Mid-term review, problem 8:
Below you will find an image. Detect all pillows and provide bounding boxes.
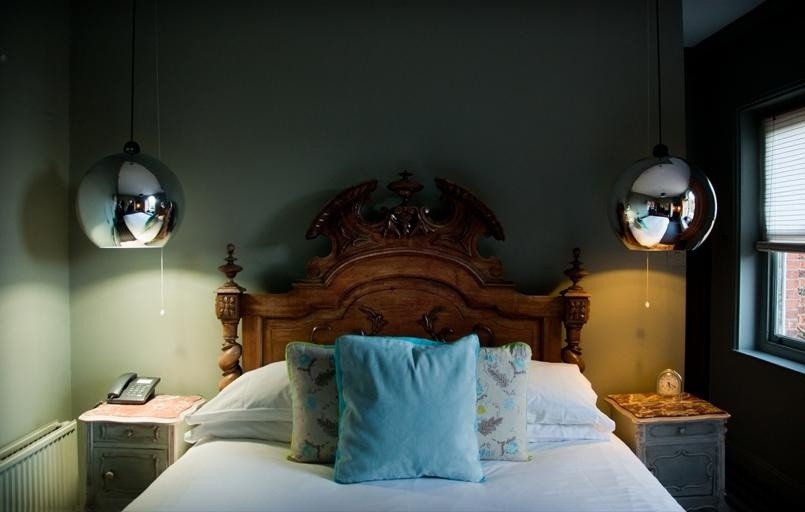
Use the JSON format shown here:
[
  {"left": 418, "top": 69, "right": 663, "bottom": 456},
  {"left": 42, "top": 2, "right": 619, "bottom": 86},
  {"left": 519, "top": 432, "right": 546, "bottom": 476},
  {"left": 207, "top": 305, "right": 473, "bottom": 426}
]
[
  {"left": 286, "top": 340, "right": 533, "bottom": 461},
  {"left": 184, "top": 423, "right": 291, "bottom": 444},
  {"left": 527, "top": 423, "right": 612, "bottom": 442},
  {"left": 183, "top": 360, "right": 294, "bottom": 425},
  {"left": 528, "top": 360, "right": 616, "bottom": 432},
  {"left": 331, "top": 333, "right": 486, "bottom": 484}
]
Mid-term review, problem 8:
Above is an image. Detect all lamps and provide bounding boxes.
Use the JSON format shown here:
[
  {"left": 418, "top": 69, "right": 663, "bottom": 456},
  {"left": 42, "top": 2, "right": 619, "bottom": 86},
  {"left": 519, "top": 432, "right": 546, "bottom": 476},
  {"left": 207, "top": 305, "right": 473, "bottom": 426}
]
[
  {"left": 607, "top": 1, "right": 717, "bottom": 252},
  {"left": 74, "top": 1, "right": 184, "bottom": 248}
]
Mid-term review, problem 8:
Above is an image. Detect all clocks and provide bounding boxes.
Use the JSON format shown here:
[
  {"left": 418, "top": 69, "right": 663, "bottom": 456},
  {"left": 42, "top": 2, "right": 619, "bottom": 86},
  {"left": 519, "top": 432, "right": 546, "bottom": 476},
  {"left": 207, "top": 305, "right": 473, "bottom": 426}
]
[{"left": 656, "top": 369, "right": 683, "bottom": 395}]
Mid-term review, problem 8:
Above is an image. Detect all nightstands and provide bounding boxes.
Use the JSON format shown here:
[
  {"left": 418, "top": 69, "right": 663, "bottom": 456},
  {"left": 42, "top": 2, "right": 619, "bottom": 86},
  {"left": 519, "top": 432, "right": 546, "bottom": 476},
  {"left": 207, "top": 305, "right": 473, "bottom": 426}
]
[
  {"left": 604, "top": 393, "right": 731, "bottom": 512},
  {"left": 78, "top": 395, "right": 206, "bottom": 512}
]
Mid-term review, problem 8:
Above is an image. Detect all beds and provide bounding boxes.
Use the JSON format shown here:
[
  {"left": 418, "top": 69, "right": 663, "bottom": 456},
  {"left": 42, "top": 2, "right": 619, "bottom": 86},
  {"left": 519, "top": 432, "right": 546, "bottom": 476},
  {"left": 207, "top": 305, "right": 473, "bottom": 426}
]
[{"left": 121, "top": 170, "right": 685, "bottom": 512}]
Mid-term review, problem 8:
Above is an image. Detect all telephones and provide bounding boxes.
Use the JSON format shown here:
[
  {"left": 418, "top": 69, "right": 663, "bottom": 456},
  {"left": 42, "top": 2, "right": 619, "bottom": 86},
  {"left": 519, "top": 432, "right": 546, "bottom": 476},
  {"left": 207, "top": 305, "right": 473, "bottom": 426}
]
[{"left": 105, "top": 372, "right": 160, "bottom": 404}]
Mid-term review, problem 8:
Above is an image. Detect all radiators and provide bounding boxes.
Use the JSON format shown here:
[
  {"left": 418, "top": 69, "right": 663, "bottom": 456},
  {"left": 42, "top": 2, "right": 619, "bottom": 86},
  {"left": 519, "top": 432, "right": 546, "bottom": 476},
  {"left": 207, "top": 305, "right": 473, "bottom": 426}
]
[{"left": 0, "top": 419, "right": 78, "bottom": 512}]
